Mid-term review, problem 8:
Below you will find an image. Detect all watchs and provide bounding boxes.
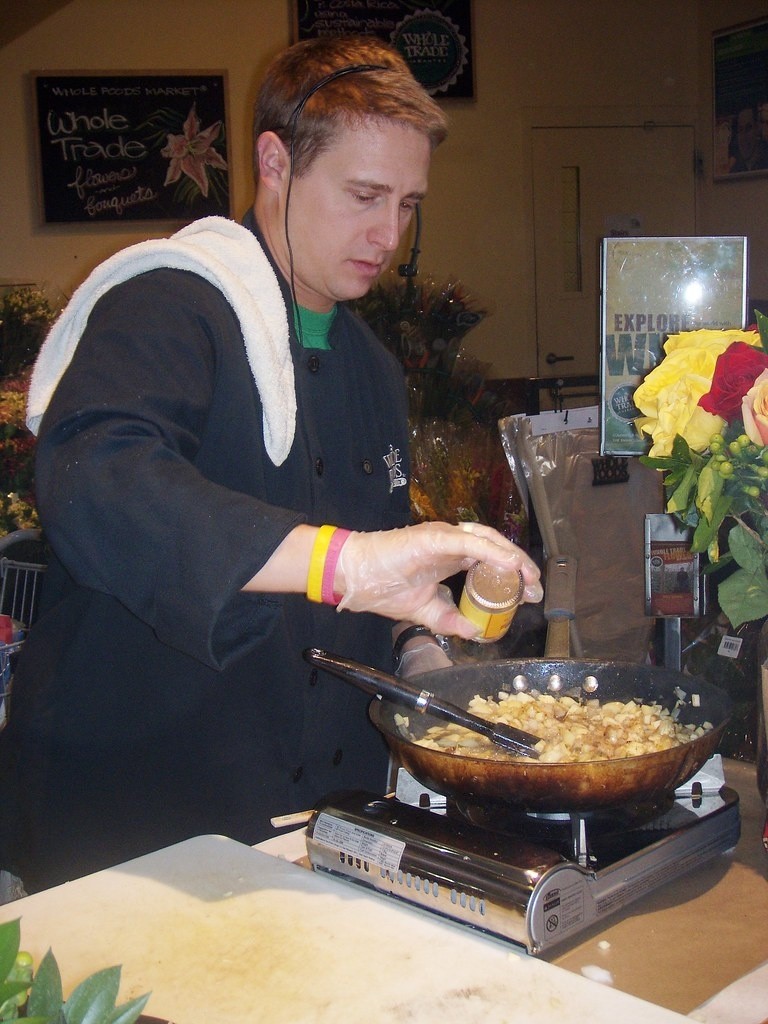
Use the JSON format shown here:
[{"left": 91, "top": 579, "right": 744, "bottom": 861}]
[{"left": 392, "top": 625, "right": 449, "bottom": 661}]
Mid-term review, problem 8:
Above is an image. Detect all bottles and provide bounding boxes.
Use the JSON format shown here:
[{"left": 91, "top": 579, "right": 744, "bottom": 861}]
[{"left": 458, "top": 559, "right": 524, "bottom": 645}]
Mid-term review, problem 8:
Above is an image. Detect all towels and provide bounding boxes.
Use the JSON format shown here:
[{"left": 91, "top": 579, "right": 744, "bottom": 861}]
[{"left": 26, "top": 214, "right": 297, "bottom": 467}]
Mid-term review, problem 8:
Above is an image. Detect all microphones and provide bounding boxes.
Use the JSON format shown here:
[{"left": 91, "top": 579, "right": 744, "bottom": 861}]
[{"left": 398, "top": 204, "right": 422, "bottom": 277}]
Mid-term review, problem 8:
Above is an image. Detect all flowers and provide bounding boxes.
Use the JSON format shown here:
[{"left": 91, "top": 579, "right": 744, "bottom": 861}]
[
  {"left": 0, "top": 278, "right": 77, "bottom": 537},
  {"left": 632, "top": 310, "right": 768, "bottom": 631},
  {"left": 348, "top": 273, "right": 528, "bottom": 550}
]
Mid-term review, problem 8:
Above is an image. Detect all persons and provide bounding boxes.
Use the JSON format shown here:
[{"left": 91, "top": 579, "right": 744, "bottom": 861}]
[{"left": 0, "top": 37, "right": 544, "bottom": 895}]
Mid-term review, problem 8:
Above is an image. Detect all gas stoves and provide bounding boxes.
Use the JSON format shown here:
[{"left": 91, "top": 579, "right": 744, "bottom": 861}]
[{"left": 305, "top": 753, "right": 742, "bottom": 956}]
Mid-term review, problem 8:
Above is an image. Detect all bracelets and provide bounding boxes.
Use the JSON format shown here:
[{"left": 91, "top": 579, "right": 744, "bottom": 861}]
[{"left": 307, "top": 525, "right": 352, "bottom": 605}]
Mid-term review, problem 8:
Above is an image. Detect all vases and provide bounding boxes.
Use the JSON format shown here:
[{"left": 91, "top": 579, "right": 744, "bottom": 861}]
[{"left": 756, "top": 620, "right": 768, "bottom": 803}]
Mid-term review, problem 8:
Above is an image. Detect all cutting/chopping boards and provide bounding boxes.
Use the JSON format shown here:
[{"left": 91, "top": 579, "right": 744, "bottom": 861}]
[{"left": 0, "top": 832, "right": 699, "bottom": 1024}]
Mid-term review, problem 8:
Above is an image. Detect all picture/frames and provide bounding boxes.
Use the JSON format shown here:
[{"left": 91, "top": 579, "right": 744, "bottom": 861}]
[{"left": 710, "top": 15, "right": 768, "bottom": 183}]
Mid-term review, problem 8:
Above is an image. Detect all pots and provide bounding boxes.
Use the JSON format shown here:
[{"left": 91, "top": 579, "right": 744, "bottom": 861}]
[{"left": 367, "top": 556, "right": 736, "bottom": 814}]
